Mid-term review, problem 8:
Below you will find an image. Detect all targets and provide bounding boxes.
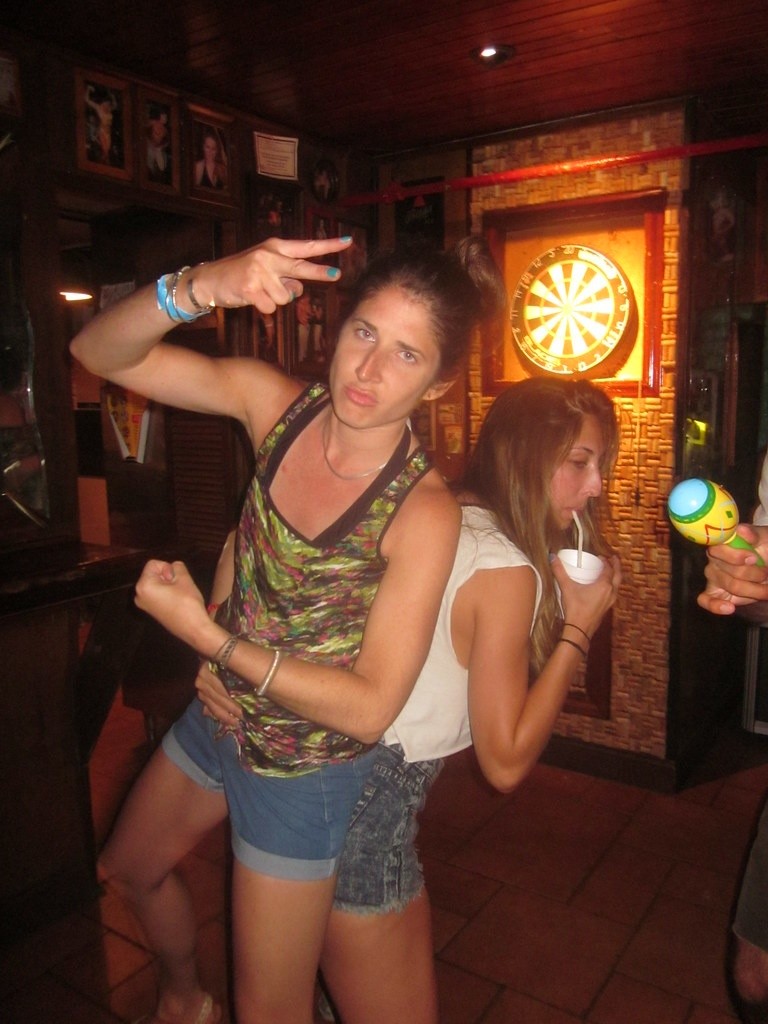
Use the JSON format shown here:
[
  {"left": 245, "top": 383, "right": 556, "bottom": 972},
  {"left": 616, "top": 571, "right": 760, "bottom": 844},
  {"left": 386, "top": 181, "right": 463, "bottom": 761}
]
[{"left": 509, "top": 241, "right": 633, "bottom": 376}]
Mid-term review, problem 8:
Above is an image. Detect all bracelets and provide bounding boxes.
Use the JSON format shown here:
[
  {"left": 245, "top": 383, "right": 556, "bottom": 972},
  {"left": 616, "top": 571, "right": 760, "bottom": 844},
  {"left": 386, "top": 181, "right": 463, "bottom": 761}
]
[
  {"left": 212, "top": 633, "right": 251, "bottom": 672},
  {"left": 252, "top": 648, "right": 284, "bottom": 698},
  {"left": 157, "top": 260, "right": 213, "bottom": 324},
  {"left": 560, "top": 622, "right": 593, "bottom": 646},
  {"left": 557, "top": 637, "right": 589, "bottom": 658}
]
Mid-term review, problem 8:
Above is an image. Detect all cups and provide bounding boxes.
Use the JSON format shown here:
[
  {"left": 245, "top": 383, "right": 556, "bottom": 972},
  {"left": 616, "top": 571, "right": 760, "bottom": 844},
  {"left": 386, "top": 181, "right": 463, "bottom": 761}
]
[{"left": 551, "top": 549, "right": 606, "bottom": 620}]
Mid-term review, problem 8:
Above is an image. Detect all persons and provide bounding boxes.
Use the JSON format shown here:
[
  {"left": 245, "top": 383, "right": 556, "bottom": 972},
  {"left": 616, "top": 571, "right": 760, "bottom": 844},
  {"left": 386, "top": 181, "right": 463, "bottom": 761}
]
[
  {"left": 84, "top": 81, "right": 126, "bottom": 168},
  {"left": 0, "top": 351, "right": 49, "bottom": 516},
  {"left": 695, "top": 440, "right": 768, "bottom": 1024},
  {"left": 252, "top": 155, "right": 421, "bottom": 374},
  {"left": 68, "top": 228, "right": 502, "bottom": 1023},
  {"left": 194, "top": 133, "right": 228, "bottom": 191},
  {"left": 145, "top": 110, "right": 174, "bottom": 178},
  {"left": 291, "top": 376, "right": 622, "bottom": 1024}
]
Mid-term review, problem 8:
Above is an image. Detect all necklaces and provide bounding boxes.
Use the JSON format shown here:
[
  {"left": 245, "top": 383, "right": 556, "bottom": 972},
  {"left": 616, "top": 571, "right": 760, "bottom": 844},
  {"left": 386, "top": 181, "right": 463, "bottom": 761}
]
[{"left": 322, "top": 401, "right": 408, "bottom": 479}]
